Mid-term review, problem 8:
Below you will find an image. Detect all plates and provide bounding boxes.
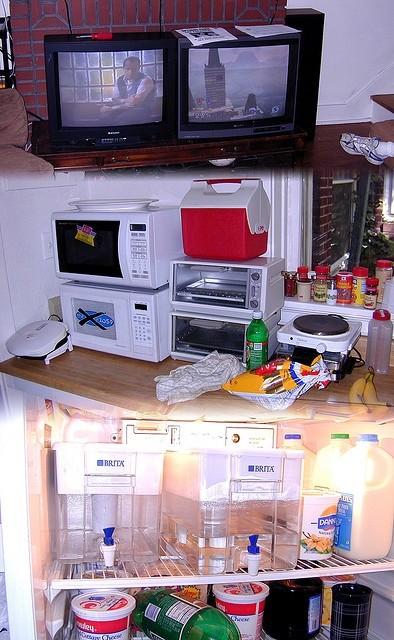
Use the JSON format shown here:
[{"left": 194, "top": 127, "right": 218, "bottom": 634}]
[{"left": 68, "top": 199, "right": 158, "bottom": 212}]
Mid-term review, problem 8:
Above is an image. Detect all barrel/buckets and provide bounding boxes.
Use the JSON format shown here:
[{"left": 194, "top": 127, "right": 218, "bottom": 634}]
[
  {"left": 331, "top": 433, "right": 394, "bottom": 561},
  {"left": 310, "top": 433, "right": 352, "bottom": 492},
  {"left": 279, "top": 435, "right": 315, "bottom": 488}
]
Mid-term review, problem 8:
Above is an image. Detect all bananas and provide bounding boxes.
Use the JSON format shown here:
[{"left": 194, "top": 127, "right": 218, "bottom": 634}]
[{"left": 349, "top": 366, "right": 390, "bottom": 415}]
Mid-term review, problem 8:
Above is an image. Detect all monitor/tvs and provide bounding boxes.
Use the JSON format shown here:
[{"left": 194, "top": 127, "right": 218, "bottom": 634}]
[
  {"left": 43, "top": 32, "right": 179, "bottom": 150},
  {"left": 171, "top": 25, "right": 301, "bottom": 141}
]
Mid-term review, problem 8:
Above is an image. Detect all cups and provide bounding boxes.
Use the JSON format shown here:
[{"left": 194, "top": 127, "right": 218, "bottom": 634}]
[{"left": 331, "top": 584, "right": 372, "bottom": 640}]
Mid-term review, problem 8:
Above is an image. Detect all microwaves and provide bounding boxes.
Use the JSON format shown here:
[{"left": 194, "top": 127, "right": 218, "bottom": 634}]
[
  {"left": 57, "top": 280, "right": 171, "bottom": 363},
  {"left": 51, "top": 206, "right": 184, "bottom": 290}
]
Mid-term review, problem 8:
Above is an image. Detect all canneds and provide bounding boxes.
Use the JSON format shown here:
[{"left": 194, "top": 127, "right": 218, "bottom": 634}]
[{"left": 313, "top": 275, "right": 326, "bottom": 302}]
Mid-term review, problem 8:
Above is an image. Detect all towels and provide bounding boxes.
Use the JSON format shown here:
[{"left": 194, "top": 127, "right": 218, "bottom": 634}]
[{"left": 155, "top": 349, "right": 243, "bottom": 406}]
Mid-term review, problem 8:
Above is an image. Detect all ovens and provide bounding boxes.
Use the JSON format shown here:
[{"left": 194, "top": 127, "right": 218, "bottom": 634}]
[
  {"left": 169, "top": 256, "right": 285, "bottom": 320},
  {"left": 167, "top": 310, "right": 281, "bottom": 367}
]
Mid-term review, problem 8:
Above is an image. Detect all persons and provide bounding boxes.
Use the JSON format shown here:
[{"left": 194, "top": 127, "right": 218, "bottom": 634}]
[{"left": 99, "top": 57, "right": 155, "bottom": 126}]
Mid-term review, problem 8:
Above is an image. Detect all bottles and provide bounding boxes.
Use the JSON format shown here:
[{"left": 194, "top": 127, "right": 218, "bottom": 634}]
[
  {"left": 314, "top": 275, "right": 327, "bottom": 302},
  {"left": 352, "top": 266, "right": 369, "bottom": 306},
  {"left": 246, "top": 311, "right": 269, "bottom": 370},
  {"left": 364, "top": 277, "right": 379, "bottom": 309},
  {"left": 365, "top": 309, "right": 393, "bottom": 374},
  {"left": 336, "top": 272, "right": 353, "bottom": 305},
  {"left": 375, "top": 260, "right": 393, "bottom": 309},
  {"left": 310, "top": 275, "right": 316, "bottom": 299},
  {"left": 284, "top": 272, "right": 296, "bottom": 296},
  {"left": 297, "top": 280, "right": 313, "bottom": 303},
  {"left": 298, "top": 266, "right": 309, "bottom": 279},
  {"left": 316, "top": 266, "right": 329, "bottom": 280},
  {"left": 327, "top": 277, "right": 338, "bottom": 304},
  {"left": 132, "top": 588, "right": 242, "bottom": 640}
]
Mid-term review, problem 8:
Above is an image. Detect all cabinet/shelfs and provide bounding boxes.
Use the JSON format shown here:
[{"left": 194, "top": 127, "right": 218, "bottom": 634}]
[
  {"left": 0, "top": 344, "right": 393, "bottom": 424},
  {"left": 372, "top": 95, "right": 393, "bottom": 179},
  {"left": 31, "top": 118, "right": 371, "bottom": 172}
]
